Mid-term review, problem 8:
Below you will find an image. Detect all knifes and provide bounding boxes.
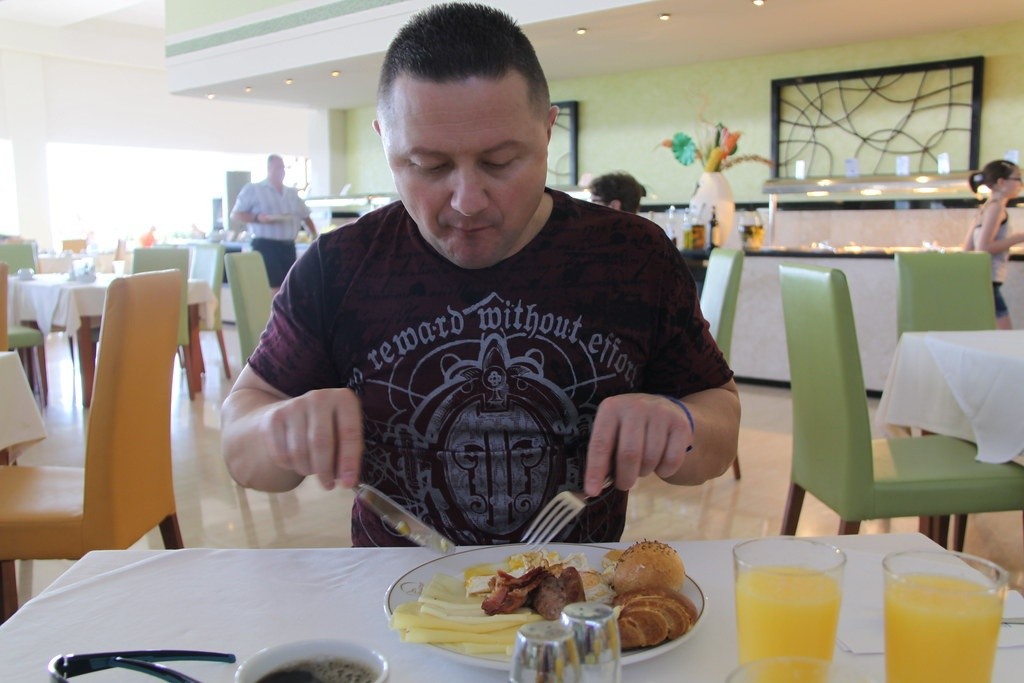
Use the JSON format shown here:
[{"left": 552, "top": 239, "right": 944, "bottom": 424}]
[{"left": 353, "top": 482, "right": 456, "bottom": 555}]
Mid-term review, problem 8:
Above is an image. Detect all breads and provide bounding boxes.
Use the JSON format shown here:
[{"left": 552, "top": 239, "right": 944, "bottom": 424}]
[{"left": 612, "top": 536, "right": 698, "bottom": 653}]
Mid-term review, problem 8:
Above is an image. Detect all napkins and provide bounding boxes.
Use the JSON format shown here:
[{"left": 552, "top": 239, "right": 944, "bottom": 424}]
[{"left": 828, "top": 586, "right": 1022, "bottom": 655}]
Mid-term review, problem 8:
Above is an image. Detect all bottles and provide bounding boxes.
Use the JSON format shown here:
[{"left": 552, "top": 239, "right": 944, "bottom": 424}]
[{"left": 666, "top": 204, "right": 720, "bottom": 250}]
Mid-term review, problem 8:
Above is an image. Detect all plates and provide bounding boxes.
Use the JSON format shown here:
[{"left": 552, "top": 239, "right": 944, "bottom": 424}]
[{"left": 384, "top": 542, "right": 705, "bottom": 671}]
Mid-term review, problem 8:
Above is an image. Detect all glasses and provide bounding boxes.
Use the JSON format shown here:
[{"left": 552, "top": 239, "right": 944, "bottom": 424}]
[
  {"left": 589, "top": 198, "right": 604, "bottom": 203},
  {"left": 1005, "top": 176, "right": 1023, "bottom": 182}
]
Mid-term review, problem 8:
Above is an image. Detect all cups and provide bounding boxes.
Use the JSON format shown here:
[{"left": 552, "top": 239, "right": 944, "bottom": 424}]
[
  {"left": 882, "top": 547, "right": 1007, "bottom": 682},
  {"left": 725, "top": 537, "right": 877, "bottom": 683},
  {"left": 738, "top": 209, "right": 765, "bottom": 249},
  {"left": 113, "top": 261, "right": 126, "bottom": 277}
]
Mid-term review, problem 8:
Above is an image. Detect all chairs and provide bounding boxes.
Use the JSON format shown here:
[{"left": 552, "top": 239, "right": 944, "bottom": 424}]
[
  {"left": 775, "top": 266, "right": 1024, "bottom": 551},
  {"left": 893, "top": 250, "right": 1001, "bottom": 344},
  {"left": 225, "top": 250, "right": 277, "bottom": 372},
  {"left": 0, "top": 265, "right": 186, "bottom": 623},
  {"left": 2, "top": 233, "right": 230, "bottom": 401},
  {"left": 700, "top": 243, "right": 744, "bottom": 481}
]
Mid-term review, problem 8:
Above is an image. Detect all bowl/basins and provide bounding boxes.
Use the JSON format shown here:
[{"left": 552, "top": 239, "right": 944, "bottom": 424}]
[{"left": 233, "top": 636, "right": 390, "bottom": 683}]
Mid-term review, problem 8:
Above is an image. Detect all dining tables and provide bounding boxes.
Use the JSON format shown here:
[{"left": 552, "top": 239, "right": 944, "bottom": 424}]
[
  {"left": 874, "top": 330, "right": 1023, "bottom": 556},
  {"left": 2, "top": 532, "right": 1022, "bottom": 683}
]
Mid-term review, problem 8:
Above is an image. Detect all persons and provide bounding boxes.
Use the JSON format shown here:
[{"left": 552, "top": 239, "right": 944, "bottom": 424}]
[
  {"left": 228, "top": 152, "right": 322, "bottom": 306},
  {"left": 584, "top": 171, "right": 648, "bottom": 218},
  {"left": 957, "top": 158, "right": 1023, "bottom": 331},
  {"left": 216, "top": 1, "right": 742, "bottom": 550}
]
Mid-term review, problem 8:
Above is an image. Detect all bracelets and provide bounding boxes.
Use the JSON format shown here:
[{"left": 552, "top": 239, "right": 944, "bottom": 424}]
[
  {"left": 658, "top": 395, "right": 696, "bottom": 435},
  {"left": 254, "top": 213, "right": 260, "bottom": 224}
]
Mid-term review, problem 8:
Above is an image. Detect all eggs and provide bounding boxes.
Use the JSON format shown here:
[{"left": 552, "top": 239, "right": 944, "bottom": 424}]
[{"left": 464, "top": 549, "right": 563, "bottom": 598}]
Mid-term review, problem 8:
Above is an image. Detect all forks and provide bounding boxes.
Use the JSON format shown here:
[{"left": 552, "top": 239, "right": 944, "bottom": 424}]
[{"left": 520, "top": 445, "right": 692, "bottom": 553}]
